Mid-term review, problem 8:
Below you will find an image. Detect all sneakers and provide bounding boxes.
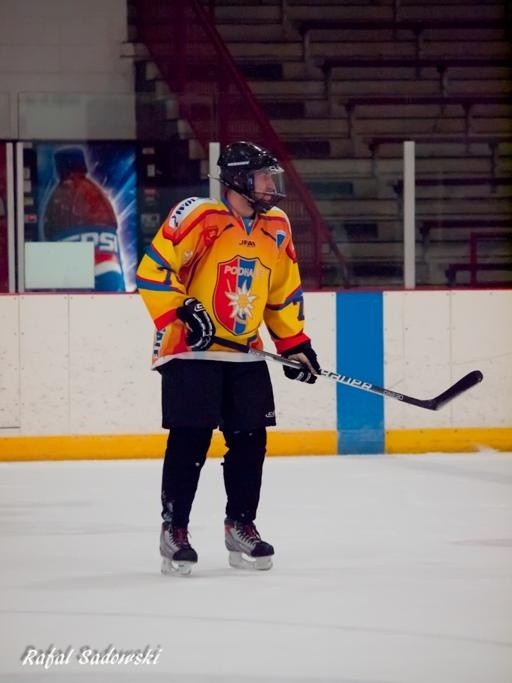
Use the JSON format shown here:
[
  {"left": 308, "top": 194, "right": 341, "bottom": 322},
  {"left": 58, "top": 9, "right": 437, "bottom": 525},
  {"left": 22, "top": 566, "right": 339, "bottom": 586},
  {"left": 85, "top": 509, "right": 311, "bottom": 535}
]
[
  {"left": 160, "top": 521, "right": 198, "bottom": 563},
  {"left": 224, "top": 518, "right": 274, "bottom": 557}
]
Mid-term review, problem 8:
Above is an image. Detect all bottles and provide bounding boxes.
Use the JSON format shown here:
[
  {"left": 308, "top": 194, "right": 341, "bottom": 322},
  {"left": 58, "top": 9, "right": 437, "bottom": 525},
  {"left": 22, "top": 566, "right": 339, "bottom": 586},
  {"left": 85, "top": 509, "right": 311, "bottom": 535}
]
[{"left": 39, "top": 145, "right": 129, "bottom": 291}]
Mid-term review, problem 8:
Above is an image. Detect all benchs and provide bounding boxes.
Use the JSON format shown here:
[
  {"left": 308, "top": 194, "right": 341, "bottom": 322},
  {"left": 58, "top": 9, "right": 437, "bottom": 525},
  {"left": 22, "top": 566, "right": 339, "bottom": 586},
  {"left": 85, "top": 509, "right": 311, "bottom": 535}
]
[{"left": 140, "top": 0, "right": 512, "bottom": 286}]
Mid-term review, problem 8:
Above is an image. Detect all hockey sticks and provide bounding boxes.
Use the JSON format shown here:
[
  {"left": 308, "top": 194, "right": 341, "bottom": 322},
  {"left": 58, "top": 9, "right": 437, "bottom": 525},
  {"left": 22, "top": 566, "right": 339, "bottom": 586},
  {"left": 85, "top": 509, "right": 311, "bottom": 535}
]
[{"left": 215, "top": 336, "right": 485, "bottom": 410}]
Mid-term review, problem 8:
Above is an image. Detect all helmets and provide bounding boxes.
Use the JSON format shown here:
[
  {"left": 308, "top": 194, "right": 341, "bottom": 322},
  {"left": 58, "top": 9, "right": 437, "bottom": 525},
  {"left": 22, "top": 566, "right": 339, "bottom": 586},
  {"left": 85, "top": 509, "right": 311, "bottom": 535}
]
[{"left": 216, "top": 141, "right": 289, "bottom": 214}]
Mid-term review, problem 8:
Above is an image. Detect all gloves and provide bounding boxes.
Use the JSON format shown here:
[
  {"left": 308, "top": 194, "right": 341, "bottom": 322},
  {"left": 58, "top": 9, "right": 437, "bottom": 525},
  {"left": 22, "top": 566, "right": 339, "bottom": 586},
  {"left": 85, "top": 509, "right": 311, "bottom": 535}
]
[
  {"left": 281, "top": 341, "right": 323, "bottom": 384},
  {"left": 175, "top": 297, "right": 215, "bottom": 352}
]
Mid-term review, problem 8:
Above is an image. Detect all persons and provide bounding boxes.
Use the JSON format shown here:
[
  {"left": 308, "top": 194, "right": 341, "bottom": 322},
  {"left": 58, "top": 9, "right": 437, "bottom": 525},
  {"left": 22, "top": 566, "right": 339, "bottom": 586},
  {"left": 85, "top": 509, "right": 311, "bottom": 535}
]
[{"left": 136, "top": 137, "right": 321, "bottom": 563}]
